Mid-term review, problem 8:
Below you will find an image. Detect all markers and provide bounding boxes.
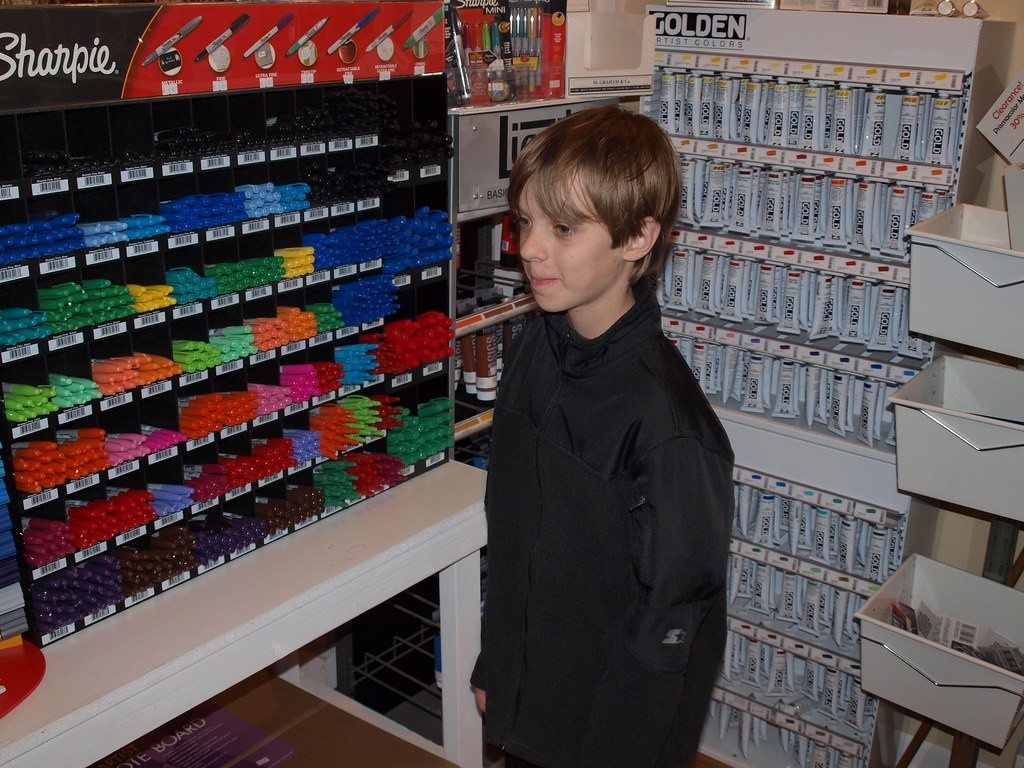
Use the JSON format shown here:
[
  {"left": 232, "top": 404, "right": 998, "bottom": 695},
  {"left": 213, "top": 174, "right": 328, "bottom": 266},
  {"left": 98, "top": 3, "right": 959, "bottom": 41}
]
[
  {"left": 1, "top": 82, "right": 462, "bottom": 646},
  {"left": 194, "top": 11, "right": 249, "bottom": 64},
  {"left": 142, "top": 14, "right": 203, "bottom": 69},
  {"left": 328, "top": 7, "right": 380, "bottom": 56},
  {"left": 284, "top": 16, "right": 330, "bottom": 57},
  {"left": 365, "top": 9, "right": 413, "bottom": 54},
  {"left": 244, "top": 12, "right": 294, "bottom": 59},
  {"left": 403, "top": 10, "right": 442, "bottom": 50}
]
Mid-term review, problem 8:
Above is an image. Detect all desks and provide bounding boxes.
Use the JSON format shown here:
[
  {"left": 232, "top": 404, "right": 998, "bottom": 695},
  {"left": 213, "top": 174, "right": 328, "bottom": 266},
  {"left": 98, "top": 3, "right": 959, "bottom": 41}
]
[{"left": 0, "top": 459, "right": 488, "bottom": 768}]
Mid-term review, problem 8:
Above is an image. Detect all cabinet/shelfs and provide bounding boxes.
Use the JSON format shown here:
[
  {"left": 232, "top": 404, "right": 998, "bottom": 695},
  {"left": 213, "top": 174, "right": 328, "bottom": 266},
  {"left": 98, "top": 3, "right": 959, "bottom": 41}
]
[
  {"left": 0, "top": 70, "right": 458, "bottom": 647},
  {"left": 646, "top": 5, "right": 1016, "bottom": 768},
  {"left": 450, "top": 93, "right": 619, "bottom": 442}
]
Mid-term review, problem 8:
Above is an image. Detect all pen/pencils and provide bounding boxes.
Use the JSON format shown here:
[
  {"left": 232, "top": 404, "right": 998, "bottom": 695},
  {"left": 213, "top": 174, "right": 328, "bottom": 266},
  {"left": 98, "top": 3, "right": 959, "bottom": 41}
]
[{"left": 449, "top": 7, "right": 549, "bottom": 102}]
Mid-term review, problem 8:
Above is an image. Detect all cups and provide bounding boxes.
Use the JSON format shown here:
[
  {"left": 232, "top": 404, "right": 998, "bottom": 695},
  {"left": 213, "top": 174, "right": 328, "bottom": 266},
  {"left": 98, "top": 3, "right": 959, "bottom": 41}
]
[{"left": 446, "top": 66, "right": 552, "bottom": 108}]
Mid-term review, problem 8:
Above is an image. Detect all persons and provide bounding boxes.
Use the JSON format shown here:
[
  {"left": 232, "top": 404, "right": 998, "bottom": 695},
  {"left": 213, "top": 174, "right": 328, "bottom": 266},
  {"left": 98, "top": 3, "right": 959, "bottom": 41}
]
[{"left": 471, "top": 106, "right": 735, "bottom": 768}]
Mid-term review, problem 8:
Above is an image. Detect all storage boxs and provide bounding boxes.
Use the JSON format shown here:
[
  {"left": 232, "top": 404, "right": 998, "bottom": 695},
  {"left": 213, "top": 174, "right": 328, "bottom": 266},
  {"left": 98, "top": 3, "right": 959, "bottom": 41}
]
[
  {"left": 855, "top": 202, "right": 1024, "bottom": 750},
  {"left": 444, "top": 0, "right": 568, "bottom": 110}
]
